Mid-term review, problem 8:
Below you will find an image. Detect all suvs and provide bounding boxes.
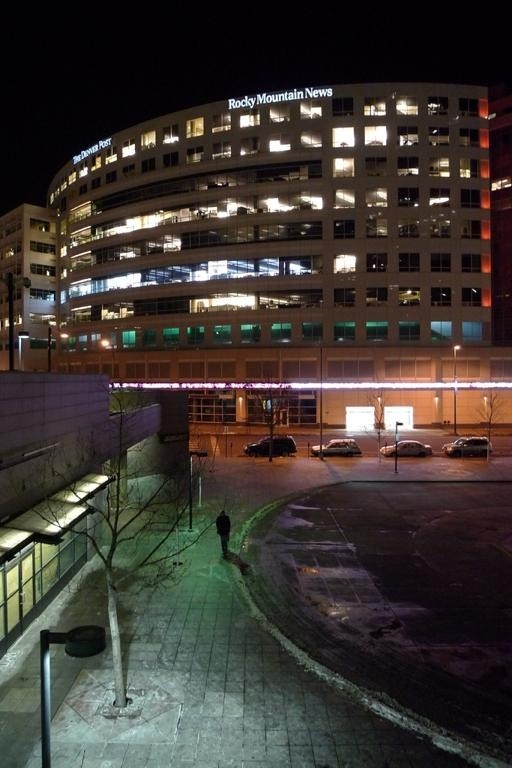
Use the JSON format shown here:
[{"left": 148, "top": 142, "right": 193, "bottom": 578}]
[
  {"left": 439, "top": 433, "right": 495, "bottom": 459},
  {"left": 242, "top": 433, "right": 298, "bottom": 458}
]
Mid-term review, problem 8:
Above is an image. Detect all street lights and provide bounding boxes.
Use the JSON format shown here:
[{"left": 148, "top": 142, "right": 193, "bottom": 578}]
[
  {"left": 37, "top": 620, "right": 112, "bottom": 765},
  {"left": 451, "top": 344, "right": 462, "bottom": 437},
  {"left": 315, "top": 335, "right": 345, "bottom": 460},
  {"left": 182, "top": 448, "right": 211, "bottom": 534},
  {"left": 393, "top": 420, "right": 403, "bottom": 473},
  {"left": 16, "top": 330, "right": 32, "bottom": 372}
]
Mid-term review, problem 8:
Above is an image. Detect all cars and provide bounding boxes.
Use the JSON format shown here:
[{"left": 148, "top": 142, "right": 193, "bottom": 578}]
[
  {"left": 380, "top": 436, "right": 433, "bottom": 459},
  {"left": 311, "top": 435, "right": 362, "bottom": 458}
]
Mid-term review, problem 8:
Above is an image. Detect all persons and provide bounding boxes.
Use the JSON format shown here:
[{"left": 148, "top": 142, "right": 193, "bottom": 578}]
[{"left": 216, "top": 511, "right": 231, "bottom": 557}]
